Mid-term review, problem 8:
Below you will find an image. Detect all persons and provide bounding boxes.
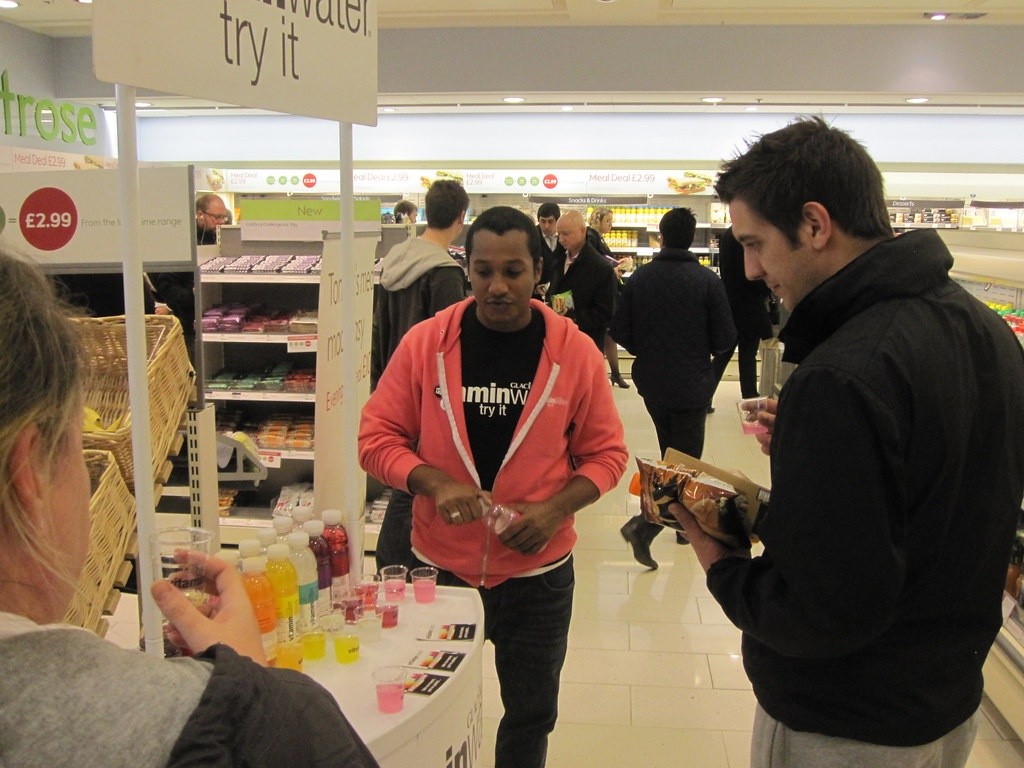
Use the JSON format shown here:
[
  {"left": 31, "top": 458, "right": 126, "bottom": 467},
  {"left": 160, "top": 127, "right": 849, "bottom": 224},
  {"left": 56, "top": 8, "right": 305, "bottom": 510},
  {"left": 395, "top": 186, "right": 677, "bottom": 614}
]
[
  {"left": 668, "top": 114, "right": 1024, "bottom": 768},
  {"left": 52, "top": 195, "right": 233, "bottom": 335},
  {"left": 719, "top": 225, "right": 780, "bottom": 399},
  {"left": 586, "top": 207, "right": 630, "bottom": 389},
  {"left": 358, "top": 206, "right": 629, "bottom": 768},
  {"left": 608, "top": 207, "right": 738, "bottom": 569},
  {"left": 0, "top": 250, "right": 382, "bottom": 768},
  {"left": 530, "top": 203, "right": 567, "bottom": 299},
  {"left": 370, "top": 180, "right": 470, "bottom": 582},
  {"left": 545, "top": 209, "right": 618, "bottom": 354},
  {"left": 393, "top": 199, "right": 417, "bottom": 224}
]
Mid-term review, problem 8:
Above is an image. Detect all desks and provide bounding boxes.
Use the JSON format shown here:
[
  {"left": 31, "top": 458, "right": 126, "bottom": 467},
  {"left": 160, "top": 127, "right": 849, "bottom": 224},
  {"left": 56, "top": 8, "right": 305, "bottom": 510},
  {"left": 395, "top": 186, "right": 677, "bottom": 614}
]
[{"left": 302, "top": 583, "right": 483, "bottom": 768}]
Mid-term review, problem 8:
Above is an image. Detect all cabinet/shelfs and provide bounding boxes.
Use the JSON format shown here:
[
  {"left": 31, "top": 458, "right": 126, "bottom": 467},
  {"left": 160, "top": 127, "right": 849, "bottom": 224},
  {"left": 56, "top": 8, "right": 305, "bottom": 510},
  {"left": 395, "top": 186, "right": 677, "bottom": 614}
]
[
  {"left": 581, "top": 200, "right": 763, "bottom": 360},
  {"left": 155, "top": 222, "right": 475, "bottom": 557}
]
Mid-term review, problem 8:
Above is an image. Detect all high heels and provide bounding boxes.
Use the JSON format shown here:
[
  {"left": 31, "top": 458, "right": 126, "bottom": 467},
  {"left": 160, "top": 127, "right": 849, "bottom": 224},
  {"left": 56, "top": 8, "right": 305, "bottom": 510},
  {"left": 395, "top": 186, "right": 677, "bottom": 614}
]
[{"left": 610, "top": 373, "right": 630, "bottom": 388}]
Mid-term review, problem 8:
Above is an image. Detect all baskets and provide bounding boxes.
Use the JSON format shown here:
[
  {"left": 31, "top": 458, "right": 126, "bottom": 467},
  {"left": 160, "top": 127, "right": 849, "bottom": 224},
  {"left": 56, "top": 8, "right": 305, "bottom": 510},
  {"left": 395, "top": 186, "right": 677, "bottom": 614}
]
[
  {"left": 60, "top": 449, "right": 137, "bottom": 632},
  {"left": 62, "top": 313, "right": 196, "bottom": 490}
]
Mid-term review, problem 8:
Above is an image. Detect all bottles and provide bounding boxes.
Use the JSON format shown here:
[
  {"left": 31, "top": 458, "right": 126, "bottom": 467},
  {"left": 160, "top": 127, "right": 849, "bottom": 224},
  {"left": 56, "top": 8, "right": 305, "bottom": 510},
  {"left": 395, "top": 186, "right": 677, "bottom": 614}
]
[
  {"left": 234, "top": 505, "right": 352, "bottom": 666},
  {"left": 477, "top": 498, "right": 547, "bottom": 555},
  {"left": 697, "top": 256, "right": 711, "bottom": 268},
  {"left": 612, "top": 254, "right": 653, "bottom": 272},
  {"left": 586, "top": 205, "right": 676, "bottom": 248},
  {"left": 980, "top": 299, "right": 1024, "bottom": 348}
]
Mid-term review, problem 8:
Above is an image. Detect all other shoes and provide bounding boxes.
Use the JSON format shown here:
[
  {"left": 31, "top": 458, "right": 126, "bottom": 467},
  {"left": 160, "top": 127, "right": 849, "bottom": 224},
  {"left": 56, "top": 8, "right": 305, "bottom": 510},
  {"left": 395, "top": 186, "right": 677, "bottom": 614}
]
[{"left": 706, "top": 406, "right": 716, "bottom": 413}]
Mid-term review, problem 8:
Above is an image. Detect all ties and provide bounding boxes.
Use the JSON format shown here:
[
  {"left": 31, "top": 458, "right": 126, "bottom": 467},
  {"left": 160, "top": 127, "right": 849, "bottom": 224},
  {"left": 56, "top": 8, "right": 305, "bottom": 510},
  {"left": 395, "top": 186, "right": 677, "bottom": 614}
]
[{"left": 547, "top": 235, "right": 556, "bottom": 252}]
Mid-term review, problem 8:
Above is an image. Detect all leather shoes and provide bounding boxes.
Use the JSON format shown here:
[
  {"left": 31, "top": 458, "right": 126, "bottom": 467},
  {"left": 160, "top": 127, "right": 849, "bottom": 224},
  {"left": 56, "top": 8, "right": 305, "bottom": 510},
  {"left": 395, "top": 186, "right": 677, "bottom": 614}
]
[
  {"left": 620, "top": 517, "right": 658, "bottom": 569},
  {"left": 677, "top": 530, "right": 689, "bottom": 545}
]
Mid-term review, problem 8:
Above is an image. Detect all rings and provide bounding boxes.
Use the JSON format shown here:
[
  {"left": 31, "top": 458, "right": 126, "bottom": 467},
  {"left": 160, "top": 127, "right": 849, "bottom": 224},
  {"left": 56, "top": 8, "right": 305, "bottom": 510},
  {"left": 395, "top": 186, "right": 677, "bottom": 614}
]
[{"left": 450, "top": 512, "right": 461, "bottom": 518}]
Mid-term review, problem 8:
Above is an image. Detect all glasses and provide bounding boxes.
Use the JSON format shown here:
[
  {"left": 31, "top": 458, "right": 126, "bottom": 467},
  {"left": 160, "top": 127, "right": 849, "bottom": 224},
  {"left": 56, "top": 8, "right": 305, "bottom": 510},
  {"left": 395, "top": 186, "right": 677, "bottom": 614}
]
[{"left": 202, "top": 210, "right": 229, "bottom": 222}]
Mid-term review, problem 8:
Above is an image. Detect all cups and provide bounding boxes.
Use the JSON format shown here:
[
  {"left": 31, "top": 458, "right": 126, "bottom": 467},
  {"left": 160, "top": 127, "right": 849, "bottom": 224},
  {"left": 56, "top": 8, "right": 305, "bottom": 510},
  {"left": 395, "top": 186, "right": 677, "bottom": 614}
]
[
  {"left": 379, "top": 565, "right": 409, "bottom": 602},
  {"left": 410, "top": 566, "right": 438, "bottom": 603},
  {"left": 372, "top": 666, "right": 408, "bottom": 714},
  {"left": 273, "top": 573, "right": 401, "bottom": 673},
  {"left": 734, "top": 395, "right": 769, "bottom": 436},
  {"left": 149, "top": 524, "right": 214, "bottom": 608}
]
